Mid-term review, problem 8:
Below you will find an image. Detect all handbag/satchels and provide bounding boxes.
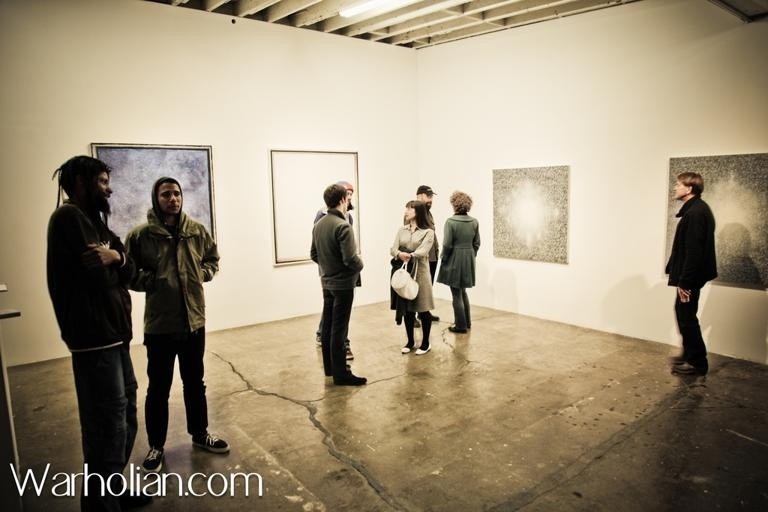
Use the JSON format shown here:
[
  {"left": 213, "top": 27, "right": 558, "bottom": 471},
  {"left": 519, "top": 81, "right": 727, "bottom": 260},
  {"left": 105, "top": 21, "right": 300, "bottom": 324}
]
[{"left": 391, "top": 253, "right": 419, "bottom": 300}]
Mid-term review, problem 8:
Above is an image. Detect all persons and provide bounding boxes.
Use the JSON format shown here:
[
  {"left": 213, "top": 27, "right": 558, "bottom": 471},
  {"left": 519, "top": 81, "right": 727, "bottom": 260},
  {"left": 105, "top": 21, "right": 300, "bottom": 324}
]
[
  {"left": 411, "top": 185, "right": 439, "bottom": 327},
  {"left": 665, "top": 170, "right": 721, "bottom": 377},
  {"left": 437, "top": 192, "right": 488, "bottom": 333},
  {"left": 311, "top": 183, "right": 373, "bottom": 386},
  {"left": 47, "top": 156, "right": 150, "bottom": 512},
  {"left": 305, "top": 181, "right": 363, "bottom": 361},
  {"left": 125, "top": 177, "right": 230, "bottom": 474},
  {"left": 391, "top": 200, "right": 435, "bottom": 355}
]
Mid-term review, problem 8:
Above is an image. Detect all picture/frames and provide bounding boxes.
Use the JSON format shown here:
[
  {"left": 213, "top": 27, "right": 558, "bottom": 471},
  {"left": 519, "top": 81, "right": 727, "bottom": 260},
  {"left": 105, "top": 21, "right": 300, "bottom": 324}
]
[
  {"left": 271, "top": 150, "right": 362, "bottom": 265},
  {"left": 90, "top": 142, "right": 215, "bottom": 246}
]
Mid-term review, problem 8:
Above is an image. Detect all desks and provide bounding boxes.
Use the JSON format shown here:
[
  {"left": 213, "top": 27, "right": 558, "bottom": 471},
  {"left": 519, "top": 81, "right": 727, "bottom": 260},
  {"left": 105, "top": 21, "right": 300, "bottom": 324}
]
[
  {"left": 0, "top": 284, "right": 11, "bottom": 295},
  {"left": 0, "top": 311, "right": 22, "bottom": 512}
]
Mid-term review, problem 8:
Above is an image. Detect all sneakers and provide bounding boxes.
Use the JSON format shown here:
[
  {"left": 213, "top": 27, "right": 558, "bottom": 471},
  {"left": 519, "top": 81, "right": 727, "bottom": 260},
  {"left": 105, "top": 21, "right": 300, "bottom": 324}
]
[
  {"left": 448, "top": 327, "right": 467, "bottom": 333},
  {"left": 346, "top": 345, "right": 354, "bottom": 360},
  {"left": 417, "top": 313, "right": 439, "bottom": 321},
  {"left": 143, "top": 446, "right": 164, "bottom": 474},
  {"left": 334, "top": 372, "right": 367, "bottom": 385},
  {"left": 192, "top": 435, "right": 229, "bottom": 453},
  {"left": 325, "top": 364, "right": 351, "bottom": 377},
  {"left": 467, "top": 325, "right": 471, "bottom": 329},
  {"left": 80, "top": 490, "right": 122, "bottom": 512},
  {"left": 316, "top": 336, "right": 322, "bottom": 347},
  {"left": 414, "top": 319, "right": 420, "bottom": 328},
  {"left": 118, "top": 485, "right": 153, "bottom": 507}
]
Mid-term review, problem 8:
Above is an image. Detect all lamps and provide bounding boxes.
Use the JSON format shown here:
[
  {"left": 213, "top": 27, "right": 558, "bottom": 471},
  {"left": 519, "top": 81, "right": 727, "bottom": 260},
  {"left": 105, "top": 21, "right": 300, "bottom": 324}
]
[{"left": 338, "top": 0, "right": 405, "bottom": 18}]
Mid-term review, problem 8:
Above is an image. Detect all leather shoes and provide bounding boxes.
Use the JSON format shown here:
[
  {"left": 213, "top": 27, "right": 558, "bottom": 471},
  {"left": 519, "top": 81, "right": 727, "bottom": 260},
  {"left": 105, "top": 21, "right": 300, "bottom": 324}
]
[
  {"left": 673, "top": 362, "right": 707, "bottom": 375},
  {"left": 670, "top": 355, "right": 686, "bottom": 365},
  {"left": 402, "top": 343, "right": 416, "bottom": 354},
  {"left": 416, "top": 344, "right": 431, "bottom": 355}
]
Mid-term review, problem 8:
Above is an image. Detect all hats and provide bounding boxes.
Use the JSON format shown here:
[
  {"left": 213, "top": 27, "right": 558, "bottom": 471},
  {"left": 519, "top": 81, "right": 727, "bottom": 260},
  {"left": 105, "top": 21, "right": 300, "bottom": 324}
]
[
  {"left": 416, "top": 185, "right": 437, "bottom": 195},
  {"left": 338, "top": 181, "right": 353, "bottom": 193}
]
